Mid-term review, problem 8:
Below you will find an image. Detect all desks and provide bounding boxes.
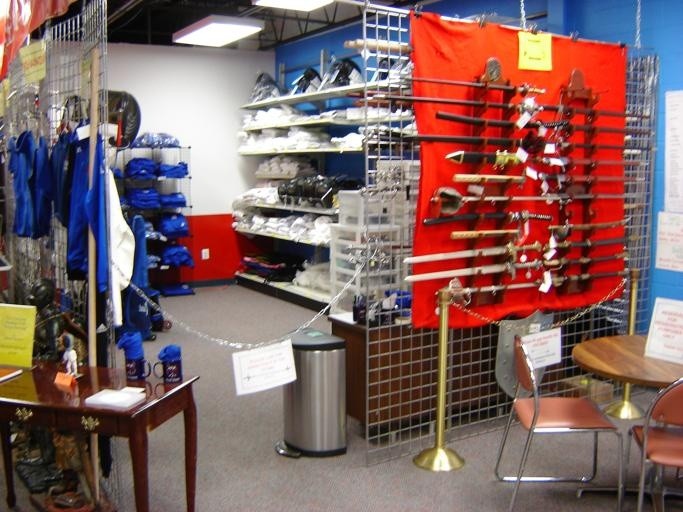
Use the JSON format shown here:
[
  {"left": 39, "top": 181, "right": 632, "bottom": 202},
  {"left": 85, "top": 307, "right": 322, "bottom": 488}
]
[
  {"left": 0, "top": 359, "right": 198, "bottom": 512},
  {"left": 570, "top": 332, "right": 682, "bottom": 511}
]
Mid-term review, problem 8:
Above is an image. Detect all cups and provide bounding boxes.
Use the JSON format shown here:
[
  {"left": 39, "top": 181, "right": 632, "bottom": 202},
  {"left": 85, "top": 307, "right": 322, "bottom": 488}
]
[
  {"left": 123, "top": 357, "right": 151, "bottom": 383},
  {"left": 152, "top": 359, "right": 182, "bottom": 383}
]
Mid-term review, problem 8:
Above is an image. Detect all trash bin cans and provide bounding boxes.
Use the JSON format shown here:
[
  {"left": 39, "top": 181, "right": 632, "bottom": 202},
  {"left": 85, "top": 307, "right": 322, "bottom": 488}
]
[{"left": 280, "top": 327, "right": 348, "bottom": 458}]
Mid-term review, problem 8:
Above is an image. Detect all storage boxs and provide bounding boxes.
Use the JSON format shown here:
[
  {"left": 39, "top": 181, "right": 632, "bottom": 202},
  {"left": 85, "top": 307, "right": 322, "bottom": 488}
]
[{"left": 559, "top": 374, "right": 615, "bottom": 405}]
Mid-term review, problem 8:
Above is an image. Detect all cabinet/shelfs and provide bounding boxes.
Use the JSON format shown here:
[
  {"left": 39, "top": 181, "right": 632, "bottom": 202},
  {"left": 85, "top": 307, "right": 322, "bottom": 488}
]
[
  {"left": 230, "top": 77, "right": 653, "bottom": 322},
  {"left": 326, "top": 303, "right": 616, "bottom": 416},
  {"left": 105, "top": 146, "right": 194, "bottom": 297}
]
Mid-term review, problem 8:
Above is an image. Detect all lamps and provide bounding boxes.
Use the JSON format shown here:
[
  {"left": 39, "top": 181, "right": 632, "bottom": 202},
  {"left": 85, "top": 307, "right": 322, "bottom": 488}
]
[
  {"left": 251, "top": 0, "right": 334, "bottom": 13},
  {"left": 171, "top": 13, "right": 265, "bottom": 49}
]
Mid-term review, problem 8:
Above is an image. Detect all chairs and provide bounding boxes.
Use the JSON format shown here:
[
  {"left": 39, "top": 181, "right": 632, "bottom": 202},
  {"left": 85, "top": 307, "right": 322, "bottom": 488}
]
[
  {"left": 492, "top": 333, "right": 623, "bottom": 511},
  {"left": 628, "top": 372, "right": 682, "bottom": 511}
]
